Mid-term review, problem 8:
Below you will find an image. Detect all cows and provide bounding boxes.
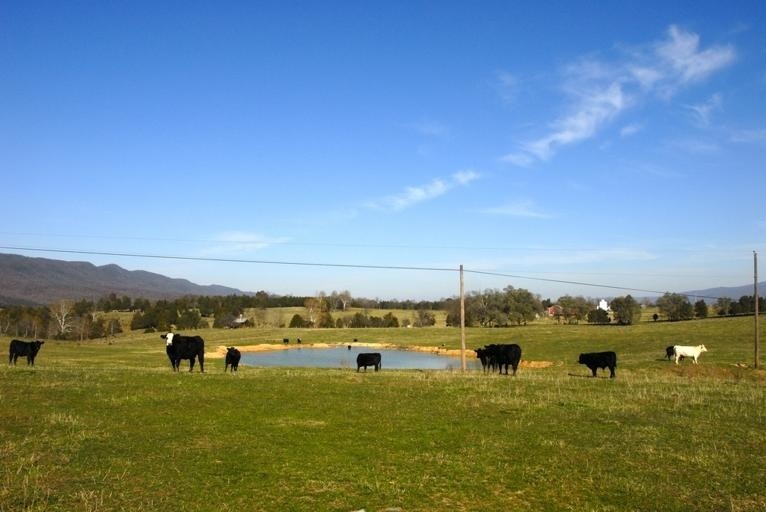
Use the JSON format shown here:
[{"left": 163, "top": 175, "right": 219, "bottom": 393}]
[
  {"left": 225, "top": 347, "right": 241, "bottom": 372},
  {"left": 579, "top": 351, "right": 617, "bottom": 378},
  {"left": 354, "top": 339, "right": 357, "bottom": 342},
  {"left": 665, "top": 346, "right": 684, "bottom": 360},
  {"left": 284, "top": 339, "right": 288, "bottom": 344},
  {"left": 674, "top": 344, "right": 708, "bottom": 364},
  {"left": 161, "top": 332, "right": 204, "bottom": 373},
  {"left": 9, "top": 340, "right": 44, "bottom": 367},
  {"left": 475, "top": 348, "right": 498, "bottom": 373},
  {"left": 357, "top": 353, "right": 381, "bottom": 371},
  {"left": 348, "top": 345, "right": 351, "bottom": 350},
  {"left": 484, "top": 344, "right": 521, "bottom": 376},
  {"left": 298, "top": 339, "right": 301, "bottom": 343}
]
